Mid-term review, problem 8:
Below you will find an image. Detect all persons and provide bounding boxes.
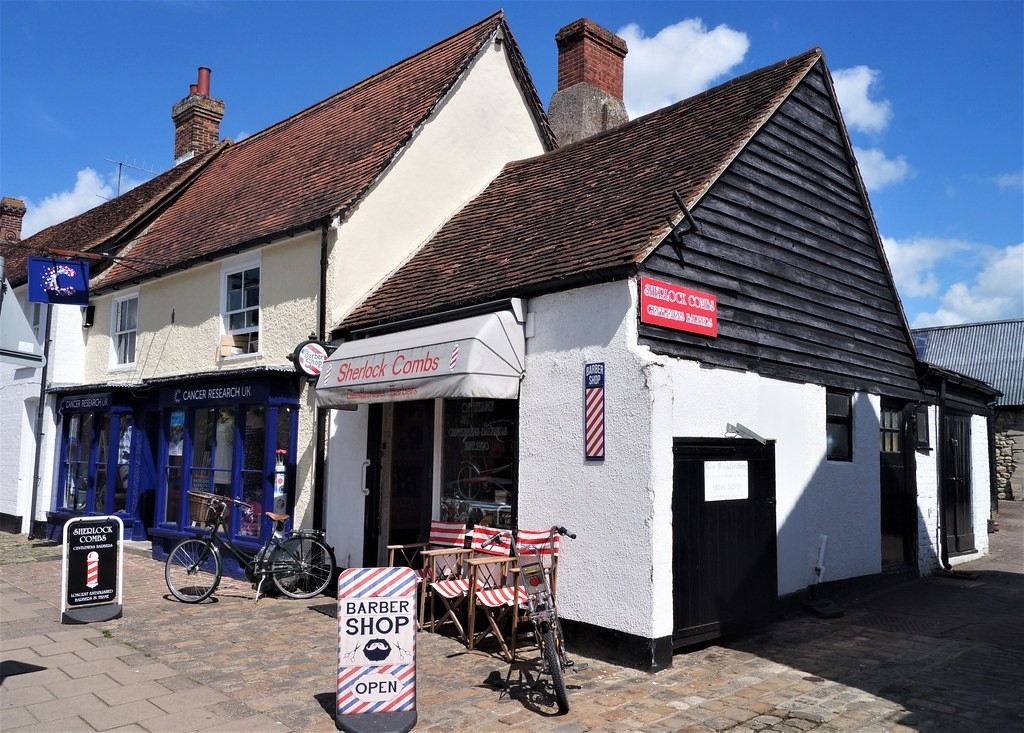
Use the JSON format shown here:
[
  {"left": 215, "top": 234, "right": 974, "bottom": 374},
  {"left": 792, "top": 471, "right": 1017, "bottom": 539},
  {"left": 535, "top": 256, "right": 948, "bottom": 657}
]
[
  {"left": 117, "top": 415, "right": 132, "bottom": 513},
  {"left": 213, "top": 406, "right": 235, "bottom": 496}
]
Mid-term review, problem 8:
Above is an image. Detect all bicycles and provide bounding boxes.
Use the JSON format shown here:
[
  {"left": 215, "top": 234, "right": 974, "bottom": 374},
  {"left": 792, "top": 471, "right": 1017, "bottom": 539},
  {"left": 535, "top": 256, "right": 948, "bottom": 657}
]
[
  {"left": 480, "top": 526, "right": 589, "bottom": 713},
  {"left": 164, "top": 489, "right": 338, "bottom": 605}
]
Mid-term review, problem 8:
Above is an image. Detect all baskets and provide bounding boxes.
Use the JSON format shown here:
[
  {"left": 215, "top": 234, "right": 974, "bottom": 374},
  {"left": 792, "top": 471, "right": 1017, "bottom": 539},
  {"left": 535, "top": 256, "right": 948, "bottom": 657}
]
[{"left": 187, "top": 490, "right": 216, "bottom": 523}]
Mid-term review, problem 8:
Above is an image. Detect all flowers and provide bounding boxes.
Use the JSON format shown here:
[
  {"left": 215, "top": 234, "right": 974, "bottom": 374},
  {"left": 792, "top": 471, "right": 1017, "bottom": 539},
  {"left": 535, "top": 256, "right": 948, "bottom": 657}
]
[{"left": 276, "top": 449, "right": 288, "bottom": 463}]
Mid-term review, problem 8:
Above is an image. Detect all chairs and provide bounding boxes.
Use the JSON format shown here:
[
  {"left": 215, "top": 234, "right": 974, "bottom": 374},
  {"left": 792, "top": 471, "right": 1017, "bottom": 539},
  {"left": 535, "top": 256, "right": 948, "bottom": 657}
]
[
  {"left": 428, "top": 524, "right": 514, "bottom": 646},
  {"left": 468, "top": 529, "right": 560, "bottom": 665},
  {"left": 382, "top": 520, "right": 467, "bottom": 633}
]
